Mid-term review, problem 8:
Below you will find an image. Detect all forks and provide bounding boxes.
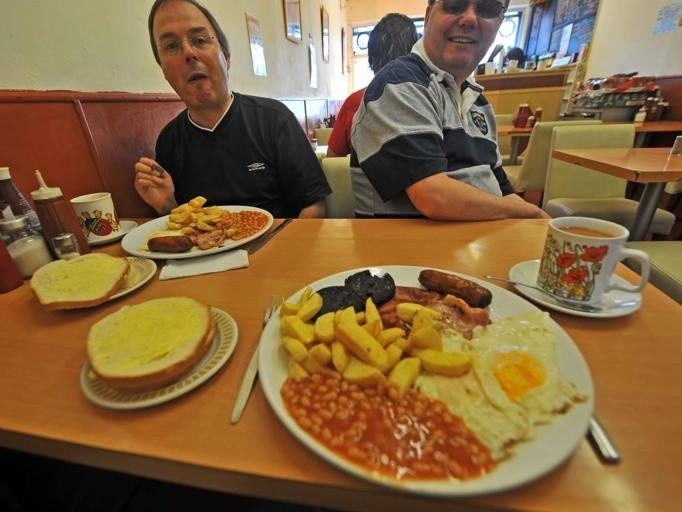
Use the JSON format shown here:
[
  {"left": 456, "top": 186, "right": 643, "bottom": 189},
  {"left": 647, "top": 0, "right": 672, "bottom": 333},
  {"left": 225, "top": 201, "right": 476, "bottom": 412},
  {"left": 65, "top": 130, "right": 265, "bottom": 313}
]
[{"left": 229, "top": 293, "right": 285, "bottom": 421}]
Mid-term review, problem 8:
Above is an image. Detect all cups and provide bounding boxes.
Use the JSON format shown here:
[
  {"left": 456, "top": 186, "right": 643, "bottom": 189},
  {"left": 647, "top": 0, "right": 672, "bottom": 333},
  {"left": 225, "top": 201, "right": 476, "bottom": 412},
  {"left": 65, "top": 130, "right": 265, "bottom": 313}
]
[
  {"left": 536, "top": 216, "right": 650, "bottom": 305},
  {"left": 70, "top": 192, "right": 120, "bottom": 241}
]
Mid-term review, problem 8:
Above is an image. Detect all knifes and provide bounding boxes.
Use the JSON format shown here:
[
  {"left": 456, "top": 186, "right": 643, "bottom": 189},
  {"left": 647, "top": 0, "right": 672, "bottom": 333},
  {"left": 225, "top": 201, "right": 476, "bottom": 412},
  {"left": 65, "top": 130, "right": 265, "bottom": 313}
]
[{"left": 248, "top": 218, "right": 292, "bottom": 255}]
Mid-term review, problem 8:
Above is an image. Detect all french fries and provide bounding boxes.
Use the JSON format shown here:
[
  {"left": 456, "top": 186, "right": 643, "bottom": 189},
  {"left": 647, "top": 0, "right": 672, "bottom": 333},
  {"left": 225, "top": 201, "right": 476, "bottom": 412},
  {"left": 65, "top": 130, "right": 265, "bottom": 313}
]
[
  {"left": 166, "top": 195, "right": 222, "bottom": 234},
  {"left": 280, "top": 286, "right": 472, "bottom": 396}
]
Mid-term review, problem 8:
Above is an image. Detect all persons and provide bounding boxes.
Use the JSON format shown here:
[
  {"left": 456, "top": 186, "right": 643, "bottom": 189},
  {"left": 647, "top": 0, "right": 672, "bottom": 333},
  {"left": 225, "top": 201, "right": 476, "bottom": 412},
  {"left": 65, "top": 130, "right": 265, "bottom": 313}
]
[
  {"left": 506, "top": 46, "right": 524, "bottom": 66},
  {"left": 350, "top": 0, "right": 556, "bottom": 223},
  {"left": 325, "top": 12, "right": 416, "bottom": 158},
  {"left": 130, "top": 0, "right": 330, "bottom": 220}
]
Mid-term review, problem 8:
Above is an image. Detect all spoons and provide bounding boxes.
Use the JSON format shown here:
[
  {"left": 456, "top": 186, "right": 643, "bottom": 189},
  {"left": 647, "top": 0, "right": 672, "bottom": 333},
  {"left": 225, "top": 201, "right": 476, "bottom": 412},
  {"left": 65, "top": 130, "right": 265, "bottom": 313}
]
[{"left": 482, "top": 273, "right": 601, "bottom": 311}]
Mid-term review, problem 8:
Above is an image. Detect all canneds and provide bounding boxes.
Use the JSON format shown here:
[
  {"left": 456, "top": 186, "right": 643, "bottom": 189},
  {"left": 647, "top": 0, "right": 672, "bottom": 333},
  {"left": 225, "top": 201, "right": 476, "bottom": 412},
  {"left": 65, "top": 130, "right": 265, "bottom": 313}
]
[{"left": 516, "top": 105, "right": 528, "bottom": 128}]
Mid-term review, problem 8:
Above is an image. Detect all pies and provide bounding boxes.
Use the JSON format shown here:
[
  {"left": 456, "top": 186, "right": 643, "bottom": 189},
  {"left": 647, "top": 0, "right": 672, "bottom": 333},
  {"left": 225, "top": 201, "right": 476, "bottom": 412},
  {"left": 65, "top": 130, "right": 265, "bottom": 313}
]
[{"left": 216, "top": 211, "right": 268, "bottom": 240}]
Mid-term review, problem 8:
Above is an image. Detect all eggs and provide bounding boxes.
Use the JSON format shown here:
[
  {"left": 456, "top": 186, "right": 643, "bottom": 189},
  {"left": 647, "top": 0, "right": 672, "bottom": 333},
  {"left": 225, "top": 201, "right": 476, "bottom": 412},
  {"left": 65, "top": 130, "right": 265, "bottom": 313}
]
[{"left": 416, "top": 310, "right": 586, "bottom": 452}]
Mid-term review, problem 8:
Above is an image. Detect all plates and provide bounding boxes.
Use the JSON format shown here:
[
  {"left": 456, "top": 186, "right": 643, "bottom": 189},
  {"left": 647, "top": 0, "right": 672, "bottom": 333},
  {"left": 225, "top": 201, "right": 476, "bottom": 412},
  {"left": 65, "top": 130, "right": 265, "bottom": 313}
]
[
  {"left": 509, "top": 256, "right": 641, "bottom": 321},
  {"left": 257, "top": 261, "right": 590, "bottom": 498},
  {"left": 106, "top": 256, "right": 158, "bottom": 301},
  {"left": 120, "top": 205, "right": 273, "bottom": 259},
  {"left": 79, "top": 306, "right": 236, "bottom": 409},
  {"left": 89, "top": 220, "right": 138, "bottom": 246}
]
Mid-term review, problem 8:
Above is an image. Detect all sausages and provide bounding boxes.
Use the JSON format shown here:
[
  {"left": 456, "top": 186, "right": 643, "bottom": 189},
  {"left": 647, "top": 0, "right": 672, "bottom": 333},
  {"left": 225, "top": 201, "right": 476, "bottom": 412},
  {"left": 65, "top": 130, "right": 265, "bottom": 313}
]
[
  {"left": 148, "top": 236, "right": 193, "bottom": 253},
  {"left": 419, "top": 270, "right": 492, "bottom": 306}
]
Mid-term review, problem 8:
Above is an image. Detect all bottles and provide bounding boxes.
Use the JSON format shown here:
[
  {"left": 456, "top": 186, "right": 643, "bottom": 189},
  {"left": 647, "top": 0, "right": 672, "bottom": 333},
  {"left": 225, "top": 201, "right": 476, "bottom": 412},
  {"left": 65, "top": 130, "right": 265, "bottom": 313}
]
[
  {"left": 0, "top": 240, "right": 24, "bottom": 293},
  {"left": 310, "top": 139, "right": 318, "bottom": 152},
  {"left": 52, "top": 233, "right": 81, "bottom": 262},
  {"left": 30, "top": 167, "right": 90, "bottom": 255},
  {"left": 1, "top": 215, "right": 52, "bottom": 278},
  {"left": 633, "top": 97, "right": 669, "bottom": 124},
  {"left": 320, "top": 118, "right": 329, "bottom": 128},
  {"left": 516, "top": 103, "right": 542, "bottom": 128},
  {"left": 1, "top": 167, "right": 59, "bottom": 260}
]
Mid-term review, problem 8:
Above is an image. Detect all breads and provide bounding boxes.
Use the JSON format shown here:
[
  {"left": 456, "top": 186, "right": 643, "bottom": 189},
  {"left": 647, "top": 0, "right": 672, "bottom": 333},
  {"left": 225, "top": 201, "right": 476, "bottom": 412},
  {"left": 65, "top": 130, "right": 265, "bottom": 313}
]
[
  {"left": 30, "top": 252, "right": 130, "bottom": 312},
  {"left": 86, "top": 296, "right": 218, "bottom": 393}
]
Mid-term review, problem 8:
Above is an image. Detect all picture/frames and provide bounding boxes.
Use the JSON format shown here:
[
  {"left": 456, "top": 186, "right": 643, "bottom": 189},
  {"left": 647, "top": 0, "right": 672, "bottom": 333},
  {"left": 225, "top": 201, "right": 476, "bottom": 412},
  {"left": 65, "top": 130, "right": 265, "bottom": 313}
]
[{"left": 246, "top": 0, "right": 348, "bottom": 89}]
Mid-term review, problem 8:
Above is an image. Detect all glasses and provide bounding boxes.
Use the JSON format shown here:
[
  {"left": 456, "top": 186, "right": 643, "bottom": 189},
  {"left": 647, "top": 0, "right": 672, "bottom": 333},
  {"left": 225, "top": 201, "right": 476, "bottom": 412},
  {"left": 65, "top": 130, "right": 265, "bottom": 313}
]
[{"left": 432, "top": 0, "right": 509, "bottom": 20}]
